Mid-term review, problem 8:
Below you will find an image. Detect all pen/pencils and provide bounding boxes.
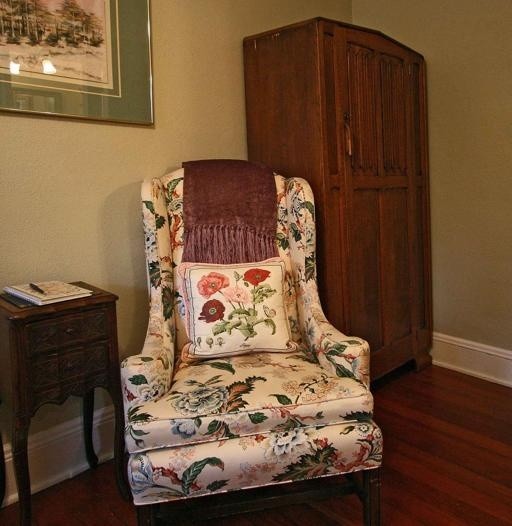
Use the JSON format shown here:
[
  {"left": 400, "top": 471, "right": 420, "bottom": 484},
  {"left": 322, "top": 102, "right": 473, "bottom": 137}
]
[{"left": 28, "top": 283, "right": 46, "bottom": 296}]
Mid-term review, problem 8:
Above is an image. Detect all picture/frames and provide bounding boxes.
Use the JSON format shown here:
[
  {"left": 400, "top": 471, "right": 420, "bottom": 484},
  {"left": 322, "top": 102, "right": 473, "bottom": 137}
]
[{"left": 0, "top": 0, "right": 154, "bottom": 128}]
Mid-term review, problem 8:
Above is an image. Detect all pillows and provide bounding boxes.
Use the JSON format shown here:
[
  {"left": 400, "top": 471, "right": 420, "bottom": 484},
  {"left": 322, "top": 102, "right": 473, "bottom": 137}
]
[{"left": 172, "top": 254, "right": 299, "bottom": 364}]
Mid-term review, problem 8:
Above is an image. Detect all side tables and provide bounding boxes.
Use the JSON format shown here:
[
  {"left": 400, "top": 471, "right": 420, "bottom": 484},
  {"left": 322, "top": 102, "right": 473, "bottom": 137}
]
[{"left": 0, "top": 279, "right": 125, "bottom": 526}]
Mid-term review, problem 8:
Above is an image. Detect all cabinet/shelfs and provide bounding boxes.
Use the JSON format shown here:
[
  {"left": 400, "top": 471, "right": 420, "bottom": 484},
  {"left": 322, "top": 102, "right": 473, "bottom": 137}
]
[{"left": 242, "top": 15, "right": 434, "bottom": 386}]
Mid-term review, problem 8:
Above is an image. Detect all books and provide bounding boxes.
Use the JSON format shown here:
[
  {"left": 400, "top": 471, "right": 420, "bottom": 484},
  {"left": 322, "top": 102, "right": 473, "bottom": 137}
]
[{"left": 2, "top": 279, "right": 93, "bottom": 307}]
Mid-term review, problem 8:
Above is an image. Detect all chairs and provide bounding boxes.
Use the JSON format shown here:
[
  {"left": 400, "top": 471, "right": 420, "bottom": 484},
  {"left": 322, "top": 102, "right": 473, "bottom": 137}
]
[{"left": 118, "top": 158, "right": 384, "bottom": 526}]
